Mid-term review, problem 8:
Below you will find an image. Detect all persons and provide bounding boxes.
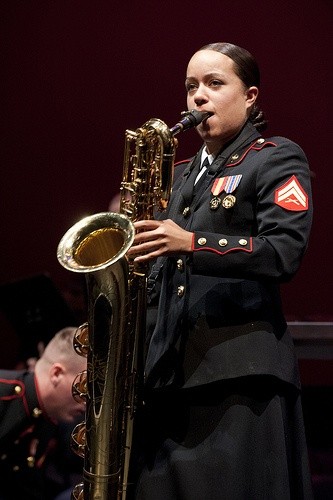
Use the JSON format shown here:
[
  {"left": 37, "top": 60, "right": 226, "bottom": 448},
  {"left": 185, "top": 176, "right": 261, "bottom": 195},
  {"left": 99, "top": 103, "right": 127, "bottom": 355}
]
[
  {"left": 126, "top": 41, "right": 313, "bottom": 500},
  {"left": 1, "top": 320, "right": 91, "bottom": 500}
]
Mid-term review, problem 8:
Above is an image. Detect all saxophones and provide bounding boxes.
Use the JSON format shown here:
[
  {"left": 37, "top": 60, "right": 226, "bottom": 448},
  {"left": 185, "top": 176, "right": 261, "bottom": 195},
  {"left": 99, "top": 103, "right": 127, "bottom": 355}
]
[{"left": 57, "top": 110, "right": 211, "bottom": 500}]
